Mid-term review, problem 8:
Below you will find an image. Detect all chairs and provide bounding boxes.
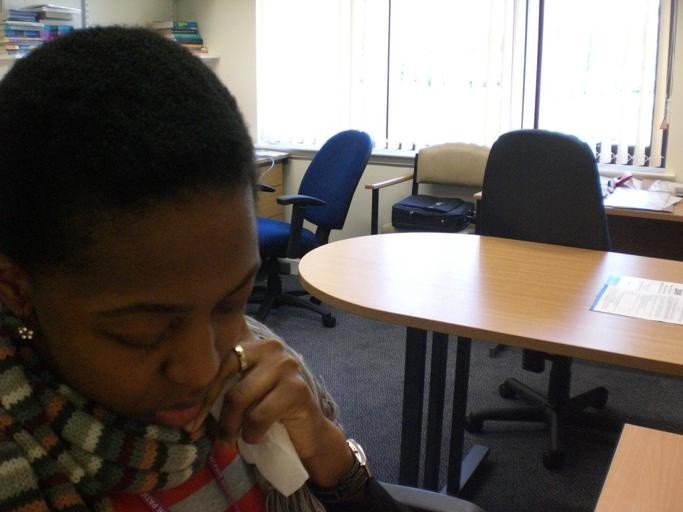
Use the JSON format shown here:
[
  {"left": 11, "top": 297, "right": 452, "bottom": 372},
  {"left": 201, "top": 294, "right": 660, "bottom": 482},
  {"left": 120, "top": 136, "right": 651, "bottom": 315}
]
[
  {"left": 365, "top": 143, "right": 490, "bottom": 235},
  {"left": 465, "top": 130, "right": 622, "bottom": 471},
  {"left": 247, "top": 130, "right": 373, "bottom": 327},
  {"left": 377, "top": 481, "right": 486, "bottom": 512}
]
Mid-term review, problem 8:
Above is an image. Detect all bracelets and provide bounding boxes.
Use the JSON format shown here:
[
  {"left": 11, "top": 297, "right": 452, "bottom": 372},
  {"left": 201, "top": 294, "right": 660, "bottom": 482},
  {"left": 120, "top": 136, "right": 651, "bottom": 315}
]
[{"left": 308, "top": 437, "right": 371, "bottom": 503}]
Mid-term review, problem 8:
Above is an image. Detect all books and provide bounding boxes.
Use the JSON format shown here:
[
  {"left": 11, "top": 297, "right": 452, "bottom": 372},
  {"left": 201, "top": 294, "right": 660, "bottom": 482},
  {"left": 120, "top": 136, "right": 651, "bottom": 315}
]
[{"left": 0, "top": 5, "right": 209, "bottom": 59}]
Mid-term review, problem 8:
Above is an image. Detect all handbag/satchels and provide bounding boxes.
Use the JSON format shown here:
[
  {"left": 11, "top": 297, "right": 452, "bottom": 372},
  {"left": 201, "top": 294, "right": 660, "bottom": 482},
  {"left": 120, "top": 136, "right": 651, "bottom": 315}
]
[{"left": 389, "top": 192, "right": 478, "bottom": 234}]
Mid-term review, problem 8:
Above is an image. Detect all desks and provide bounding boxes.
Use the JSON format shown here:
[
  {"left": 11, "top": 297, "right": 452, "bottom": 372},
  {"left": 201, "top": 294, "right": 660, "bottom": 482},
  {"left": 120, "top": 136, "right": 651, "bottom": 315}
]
[
  {"left": 603, "top": 194, "right": 683, "bottom": 222},
  {"left": 594, "top": 423, "right": 683, "bottom": 512},
  {"left": 298, "top": 232, "right": 683, "bottom": 497}
]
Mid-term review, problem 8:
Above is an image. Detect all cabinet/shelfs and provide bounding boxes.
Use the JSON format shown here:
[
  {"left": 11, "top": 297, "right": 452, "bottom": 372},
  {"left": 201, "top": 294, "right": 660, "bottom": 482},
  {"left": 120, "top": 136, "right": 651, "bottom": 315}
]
[{"left": 254, "top": 151, "right": 289, "bottom": 222}]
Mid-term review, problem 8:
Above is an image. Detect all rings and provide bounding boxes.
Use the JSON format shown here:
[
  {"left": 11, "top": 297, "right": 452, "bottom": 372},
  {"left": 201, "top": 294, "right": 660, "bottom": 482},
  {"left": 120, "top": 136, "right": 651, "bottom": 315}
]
[{"left": 231, "top": 345, "right": 250, "bottom": 373}]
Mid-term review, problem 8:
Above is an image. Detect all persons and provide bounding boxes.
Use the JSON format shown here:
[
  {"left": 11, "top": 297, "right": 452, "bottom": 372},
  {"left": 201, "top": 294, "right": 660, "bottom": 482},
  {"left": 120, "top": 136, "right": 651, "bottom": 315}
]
[{"left": 0, "top": 25, "right": 402, "bottom": 511}]
